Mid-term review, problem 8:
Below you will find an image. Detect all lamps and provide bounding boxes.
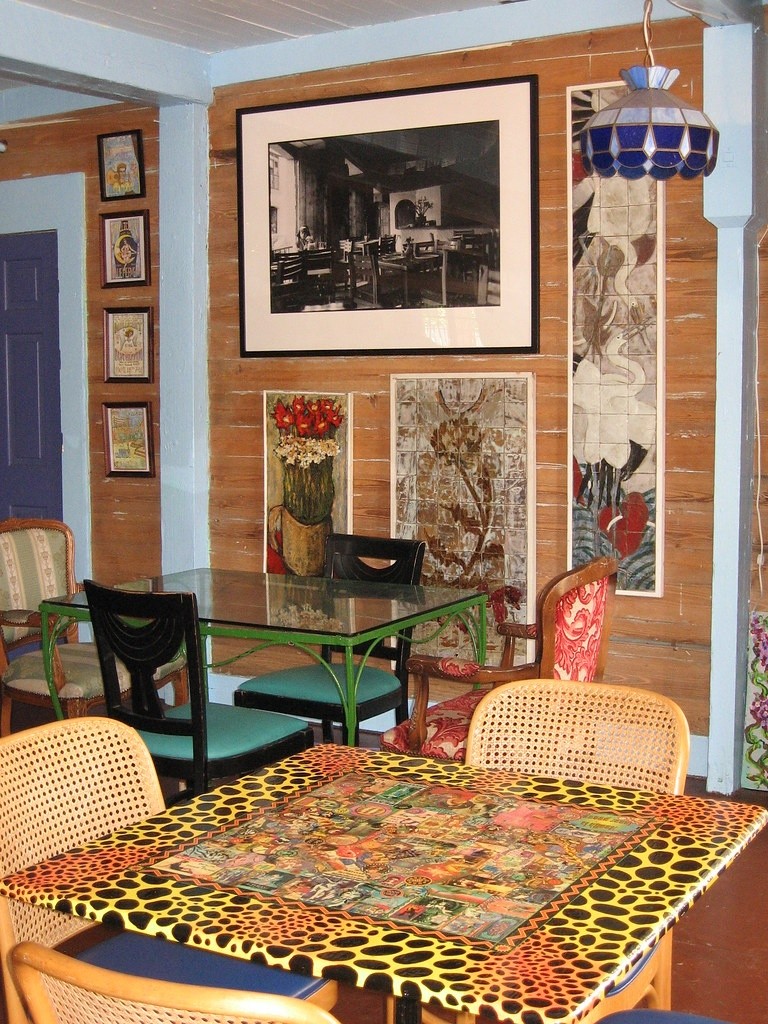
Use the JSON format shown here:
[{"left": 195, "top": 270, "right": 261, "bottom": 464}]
[{"left": 578, "top": 0, "right": 722, "bottom": 182}]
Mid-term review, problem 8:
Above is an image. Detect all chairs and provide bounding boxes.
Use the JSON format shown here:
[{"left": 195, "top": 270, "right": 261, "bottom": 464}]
[{"left": 0, "top": 514, "right": 692, "bottom": 1024}]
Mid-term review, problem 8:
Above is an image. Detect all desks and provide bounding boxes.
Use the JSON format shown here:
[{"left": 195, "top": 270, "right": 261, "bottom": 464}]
[
  {"left": 0, "top": 745, "right": 768, "bottom": 1024},
  {"left": 37, "top": 566, "right": 493, "bottom": 749}
]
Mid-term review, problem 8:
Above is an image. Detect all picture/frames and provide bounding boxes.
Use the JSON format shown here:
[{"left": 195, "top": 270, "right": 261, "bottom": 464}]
[
  {"left": 102, "top": 307, "right": 156, "bottom": 385},
  {"left": 266, "top": 574, "right": 357, "bottom": 636},
  {"left": 98, "top": 209, "right": 152, "bottom": 290},
  {"left": 101, "top": 401, "right": 156, "bottom": 479},
  {"left": 564, "top": 80, "right": 668, "bottom": 601},
  {"left": 389, "top": 370, "right": 535, "bottom": 672},
  {"left": 261, "top": 388, "right": 355, "bottom": 574},
  {"left": 97, "top": 128, "right": 147, "bottom": 202},
  {"left": 237, "top": 73, "right": 541, "bottom": 360}
]
